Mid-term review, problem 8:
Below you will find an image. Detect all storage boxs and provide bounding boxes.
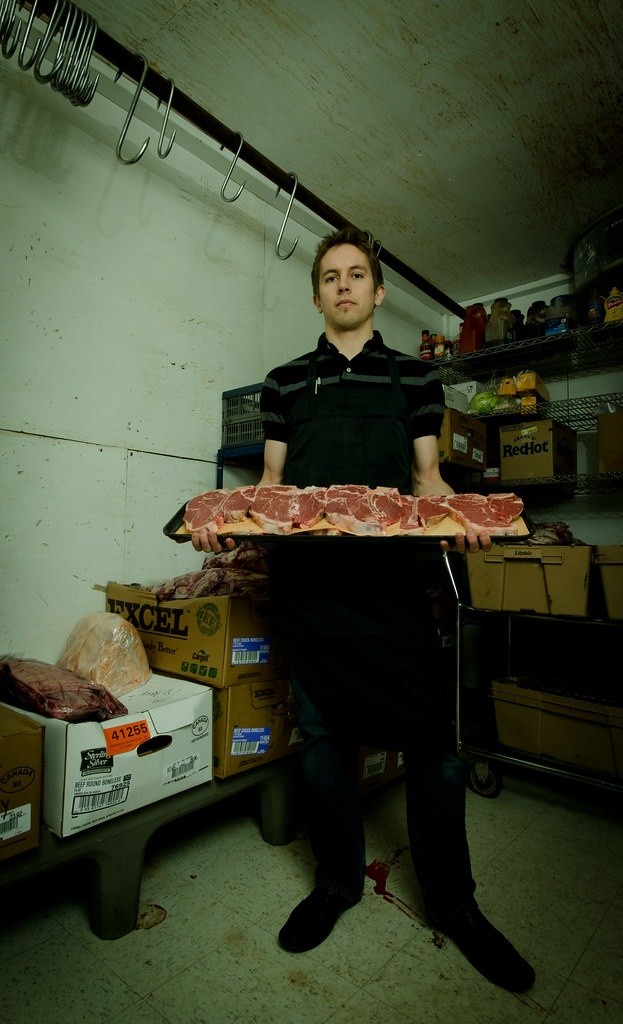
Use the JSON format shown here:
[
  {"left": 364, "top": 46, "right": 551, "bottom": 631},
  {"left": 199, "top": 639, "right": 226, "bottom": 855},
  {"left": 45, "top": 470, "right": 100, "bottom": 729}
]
[
  {"left": 101, "top": 581, "right": 289, "bottom": 688},
  {"left": 221, "top": 382, "right": 266, "bottom": 449},
  {"left": 432, "top": 406, "right": 623, "bottom": 780},
  {"left": 359, "top": 746, "right": 409, "bottom": 795},
  {"left": 215, "top": 678, "right": 294, "bottom": 781},
  {"left": 0, "top": 672, "right": 214, "bottom": 841},
  {"left": 0, "top": 705, "right": 44, "bottom": 861}
]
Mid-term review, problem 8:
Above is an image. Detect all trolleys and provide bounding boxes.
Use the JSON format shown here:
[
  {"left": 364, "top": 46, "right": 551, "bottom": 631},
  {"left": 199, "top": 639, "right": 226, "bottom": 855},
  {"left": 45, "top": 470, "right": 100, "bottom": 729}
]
[{"left": 442, "top": 550, "right": 622, "bottom": 801}]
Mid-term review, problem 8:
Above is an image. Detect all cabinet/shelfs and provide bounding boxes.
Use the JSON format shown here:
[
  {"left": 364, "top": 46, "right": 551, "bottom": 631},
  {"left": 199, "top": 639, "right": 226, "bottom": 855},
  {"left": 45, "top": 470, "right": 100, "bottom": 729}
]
[{"left": 417, "top": 314, "right": 623, "bottom": 800}]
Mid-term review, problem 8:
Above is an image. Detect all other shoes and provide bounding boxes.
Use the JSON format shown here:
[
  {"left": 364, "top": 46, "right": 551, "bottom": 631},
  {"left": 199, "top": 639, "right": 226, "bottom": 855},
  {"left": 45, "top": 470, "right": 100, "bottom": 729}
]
[
  {"left": 426, "top": 904, "right": 535, "bottom": 993},
  {"left": 278, "top": 887, "right": 362, "bottom": 952}
]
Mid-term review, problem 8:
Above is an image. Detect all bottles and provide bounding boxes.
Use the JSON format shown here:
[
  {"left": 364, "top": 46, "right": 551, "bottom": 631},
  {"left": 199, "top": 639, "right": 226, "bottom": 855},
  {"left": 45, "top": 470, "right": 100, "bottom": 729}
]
[
  {"left": 525, "top": 301, "right": 549, "bottom": 336},
  {"left": 451, "top": 323, "right": 463, "bottom": 356},
  {"left": 420, "top": 330, "right": 451, "bottom": 359},
  {"left": 460, "top": 303, "right": 487, "bottom": 353},
  {"left": 486, "top": 298, "right": 517, "bottom": 346},
  {"left": 600, "top": 287, "right": 623, "bottom": 323},
  {"left": 587, "top": 300, "right": 601, "bottom": 325}
]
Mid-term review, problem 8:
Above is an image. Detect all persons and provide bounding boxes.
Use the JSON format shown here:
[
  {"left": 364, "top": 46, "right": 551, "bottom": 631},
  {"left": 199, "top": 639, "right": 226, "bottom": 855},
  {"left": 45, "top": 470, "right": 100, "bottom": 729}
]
[{"left": 192, "top": 225, "right": 536, "bottom": 992}]
